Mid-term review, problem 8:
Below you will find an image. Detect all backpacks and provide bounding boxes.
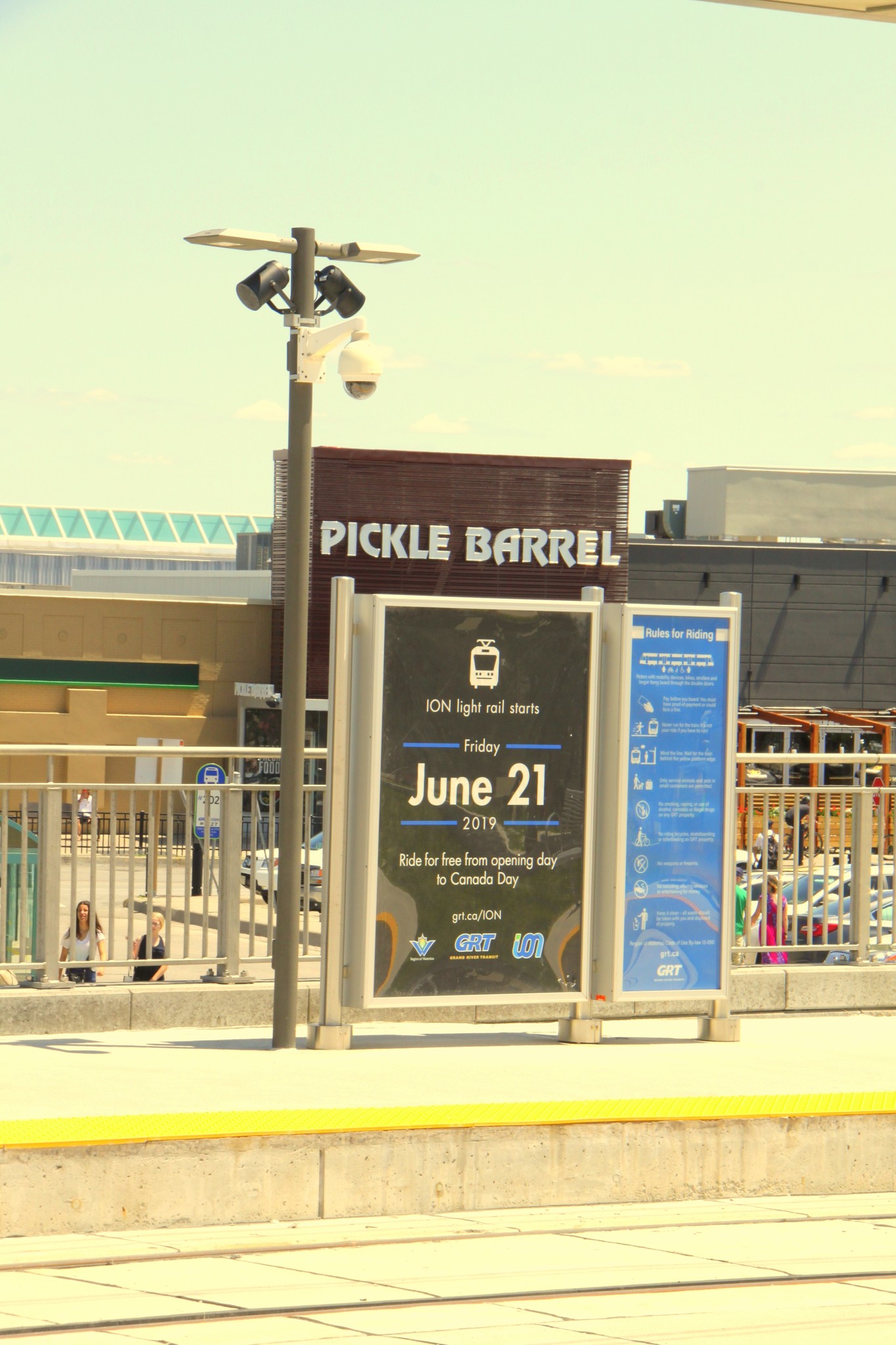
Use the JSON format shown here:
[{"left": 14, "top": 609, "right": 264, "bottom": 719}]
[{"left": 758, "top": 832, "right": 779, "bottom": 870}]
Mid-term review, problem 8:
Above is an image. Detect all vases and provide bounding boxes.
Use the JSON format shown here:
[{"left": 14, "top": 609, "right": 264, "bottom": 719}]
[{"left": 744, "top": 814, "right": 892, "bottom": 851}]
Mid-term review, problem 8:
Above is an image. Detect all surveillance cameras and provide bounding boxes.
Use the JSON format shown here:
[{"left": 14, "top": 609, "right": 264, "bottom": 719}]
[
  {"left": 266, "top": 699, "right": 280, "bottom": 708},
  {"left": 338, "top": 331, "right": 382, "bottom": 400}
]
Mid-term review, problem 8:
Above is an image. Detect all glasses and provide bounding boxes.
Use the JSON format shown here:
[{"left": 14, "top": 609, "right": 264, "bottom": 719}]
[{"left": 152, "top": 922, "right": 161, "bottom": 927}]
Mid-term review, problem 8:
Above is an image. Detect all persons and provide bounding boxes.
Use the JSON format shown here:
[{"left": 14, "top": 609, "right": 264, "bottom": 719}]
[
  {"left": 77, "top": 789, "right": 92, "bottom": 841},
  {"left": 753, "top": 820, "right": 779, "bottom": 870},
  {"left": 123, "top": 912, "right": 169, "bottom": 982},
  {"left": 785, "top": 795, "right": 810, "bottom": 844},
  {"left": 59, "top": 901, "right": 107, "bottom": 982},
  {"left": 731, "top": 867, "right": 747, "bottom": 966},
  {"left": 751, "top": 875, "right": 788, "bottom": 965}
]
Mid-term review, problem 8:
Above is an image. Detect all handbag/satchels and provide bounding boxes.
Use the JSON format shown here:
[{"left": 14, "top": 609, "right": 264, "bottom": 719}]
[
  {"left": 63, "top": 972, "right": 88, "bottom": 983},
  {"left": 123, "top": 966, "right": 133, "bottom": 981}
]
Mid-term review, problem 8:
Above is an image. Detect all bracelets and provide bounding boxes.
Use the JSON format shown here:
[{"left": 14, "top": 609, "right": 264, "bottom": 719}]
[{"left": 784, "top": 933, "right": 787, "bottom": 935}]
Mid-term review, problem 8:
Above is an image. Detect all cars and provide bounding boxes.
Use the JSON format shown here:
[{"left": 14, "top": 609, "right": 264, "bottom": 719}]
[
  {"left": 242, "top": 829, "right": 322, "bottom": 911},
  {"left": 735, "top": 848, "right": 895, "bottom": 964}
]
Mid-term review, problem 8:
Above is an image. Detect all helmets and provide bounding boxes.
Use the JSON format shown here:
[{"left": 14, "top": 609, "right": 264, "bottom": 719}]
[{"left": 800, "top": 796, "right": 810, "bottom": 804}]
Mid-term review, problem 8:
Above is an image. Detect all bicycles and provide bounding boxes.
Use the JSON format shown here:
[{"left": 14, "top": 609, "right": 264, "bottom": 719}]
[{"left": 782, "top": 822, "right": 824, "bottom": 860}]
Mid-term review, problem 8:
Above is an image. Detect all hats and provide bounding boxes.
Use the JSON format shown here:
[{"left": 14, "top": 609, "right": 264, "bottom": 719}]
[{"left": 736, "top": 867, "right": 744, "bottom": 879}]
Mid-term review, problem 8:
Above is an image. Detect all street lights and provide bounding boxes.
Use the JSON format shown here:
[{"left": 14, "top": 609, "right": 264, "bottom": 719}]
[{"left": 178, "top": 225, "right": 436, "bottom": 1051}]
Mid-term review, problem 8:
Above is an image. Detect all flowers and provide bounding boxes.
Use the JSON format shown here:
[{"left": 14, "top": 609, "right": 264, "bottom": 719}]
[{"left": 747, "top": 805, "right": 854, "bottom": 817}]
